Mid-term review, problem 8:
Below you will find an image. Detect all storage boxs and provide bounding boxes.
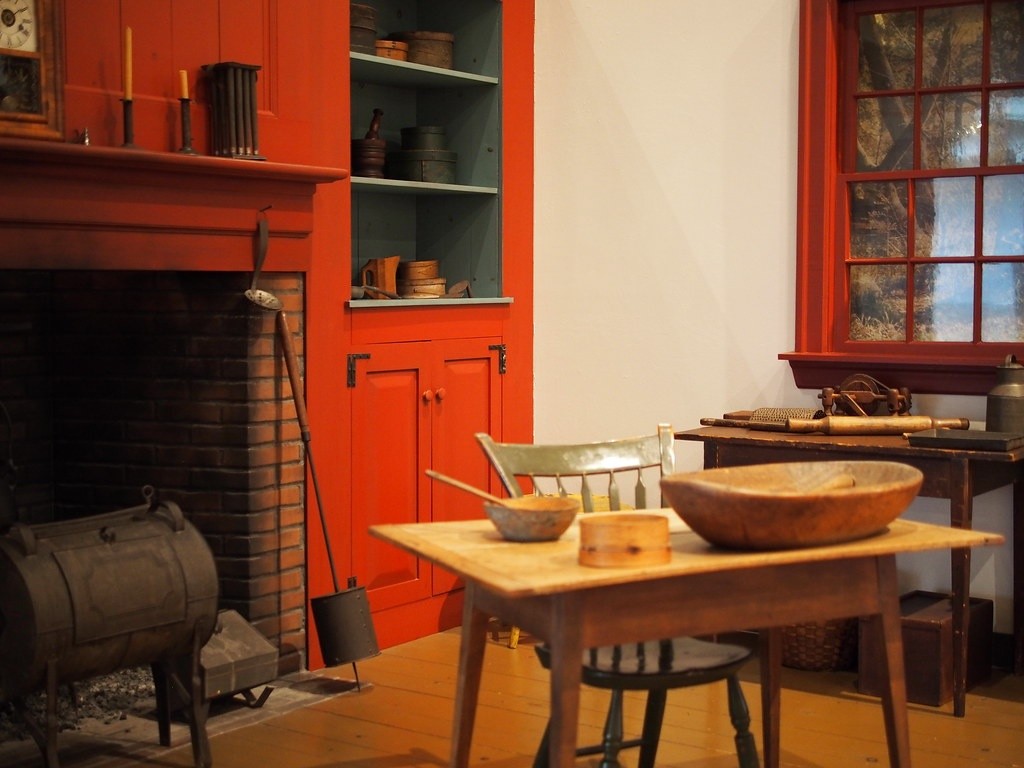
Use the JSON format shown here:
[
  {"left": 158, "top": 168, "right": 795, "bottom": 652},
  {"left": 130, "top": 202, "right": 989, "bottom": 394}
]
[{"left": 856, "top": 590, "right": 994, "bottom": 706}]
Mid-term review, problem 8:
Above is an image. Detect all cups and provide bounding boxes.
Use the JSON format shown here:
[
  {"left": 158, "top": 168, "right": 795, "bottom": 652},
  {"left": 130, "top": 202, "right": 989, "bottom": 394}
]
[{"left": 353, "top": 138, "right": 386, "bottom": 177}]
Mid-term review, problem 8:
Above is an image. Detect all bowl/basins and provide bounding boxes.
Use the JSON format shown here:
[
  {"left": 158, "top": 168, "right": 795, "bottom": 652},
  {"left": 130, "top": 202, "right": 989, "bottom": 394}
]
[
  {"left": 579, "top": 514, "right": 673, "bottom": 565},
  {"left": 658, "top": 460, "right": 923, "bottom": 549},
  {"left": 484, "top": 496, "right": 581, "bottom": 541}
]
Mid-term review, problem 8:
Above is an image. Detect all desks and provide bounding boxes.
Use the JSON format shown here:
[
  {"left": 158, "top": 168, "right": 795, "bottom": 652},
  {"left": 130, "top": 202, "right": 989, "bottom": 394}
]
[
  {"left": 367, "top": 508, "right": 1004, "bottom": 768},
  {"left": 674, "top": 423, "right": 1024, "bottom": 715}
]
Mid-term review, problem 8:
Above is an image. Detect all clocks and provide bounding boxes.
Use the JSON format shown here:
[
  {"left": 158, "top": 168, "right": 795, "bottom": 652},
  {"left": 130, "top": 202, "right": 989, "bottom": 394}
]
[{"left": 0, "top": 0, "right": 66, "bottom": 139}]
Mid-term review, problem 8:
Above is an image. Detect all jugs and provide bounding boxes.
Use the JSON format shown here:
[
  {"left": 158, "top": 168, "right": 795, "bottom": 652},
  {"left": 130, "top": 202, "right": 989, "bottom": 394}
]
[{"left": 362, "top": 255, "right": 400, "bottom": 298}]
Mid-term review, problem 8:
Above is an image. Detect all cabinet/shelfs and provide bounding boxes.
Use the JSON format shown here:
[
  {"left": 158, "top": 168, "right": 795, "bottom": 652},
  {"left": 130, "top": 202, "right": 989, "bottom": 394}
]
[{"left": 310, "top": 0, "right": 534, "bottom": 668}]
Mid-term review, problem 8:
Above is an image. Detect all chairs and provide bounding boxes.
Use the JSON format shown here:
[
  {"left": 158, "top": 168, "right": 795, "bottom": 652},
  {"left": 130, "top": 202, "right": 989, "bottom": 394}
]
[{"left": 475, "top": 423, "right": 759, "bottom": 768}]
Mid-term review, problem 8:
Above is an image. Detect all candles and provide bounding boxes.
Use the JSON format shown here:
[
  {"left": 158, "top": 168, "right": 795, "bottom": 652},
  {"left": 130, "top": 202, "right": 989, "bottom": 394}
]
[
  {"left": 124, "top": 26, "right": 133, "bottom": 100},
  {"left": 179, "top": 69, "right": 189, "bottom": 97}
]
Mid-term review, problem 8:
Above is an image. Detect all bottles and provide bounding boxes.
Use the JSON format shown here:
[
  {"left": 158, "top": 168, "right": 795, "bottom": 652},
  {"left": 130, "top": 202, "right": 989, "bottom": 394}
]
[{"left": 986, "top": 353, "right": 1024, "bottom": 433}]
